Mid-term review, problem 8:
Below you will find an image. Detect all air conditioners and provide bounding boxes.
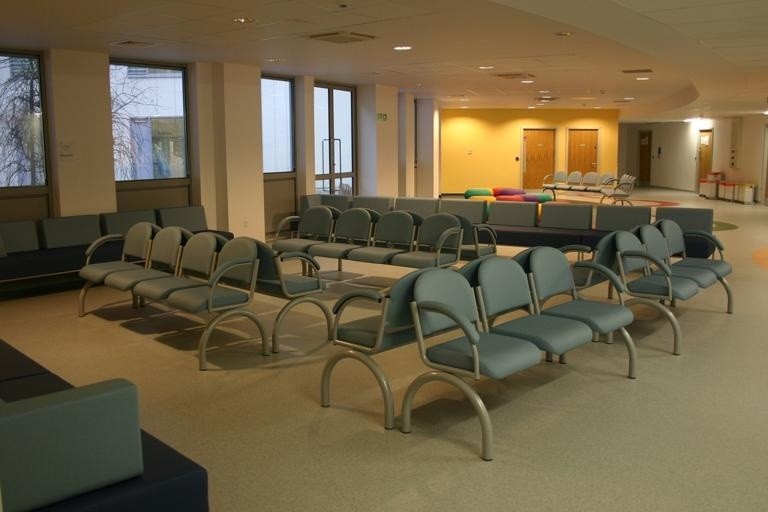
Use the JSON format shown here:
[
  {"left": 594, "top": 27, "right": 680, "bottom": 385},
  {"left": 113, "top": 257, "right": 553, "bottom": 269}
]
[{"left": 312, "top": 30, "right": 373, "bottom": 44}]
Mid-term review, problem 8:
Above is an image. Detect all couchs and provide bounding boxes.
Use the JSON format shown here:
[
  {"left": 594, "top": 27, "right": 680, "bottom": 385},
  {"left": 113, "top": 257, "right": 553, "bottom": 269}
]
[
  {"left": 40, "top": 213, "right": 102, "bottom": 294},
  {"left": 0, "top": 337, "right": 209, "bottom": 512},
  {"left": 655, "top": 204, "right": 717, "bottom": 261},
  {"left": 541, "top": 202, "right": 593, "bottom": 262},
  {"left": 101, "top": 211, "right": 158, "bottom": 267},
  {"left": 488, "top": 199, "right": 538, "bottom": 247},
  {"left": 439, "top": 198, "right": 487, "bottom": 244},
  {"left": 320, "top": 194, "right": 349, "bottom": 213},
  {"left": 595, "top": 204, "right": 651, "bottom": 249},
  {"left": 300, "top": 194, "right": 322, "bottom": 217},
  {"left": 156, "top": 207, "right": 234, "bottom": 244},
  {"left": 395, "top": 198, "right": 439, "bottom": 218},
  {"left": 351, "top": 195, "right": 393, "bottom": 216},
  {"left": 0, "top": 219, "right": 40, "bottom": 300}
]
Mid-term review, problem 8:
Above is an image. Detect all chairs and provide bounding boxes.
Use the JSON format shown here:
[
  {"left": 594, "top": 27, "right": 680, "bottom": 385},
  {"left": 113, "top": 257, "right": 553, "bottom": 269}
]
[
  {"left": 615, "top": 220, "right": 698, "bottom": 354},
  {"left": 541, "top": 171, "right": 567, "bottom": 202},
  {"left": 392, "top": 213, "right": 463, "bottom": 268},
  {"left": 457, "top": 254, "right": 497, "bottom": 286},
  {"left": 272, "top": 207, "right": 333, "bottom": 276},
  {"left": 640, "top": 223, "right": 717, "bottom": 304},
  {"left": 347, "top": 211, "right": 414, "bottom": 265},
  {"left": 587, "top": 174, "right": 614, "bottom": 193},
  {"left": 559, "top": 231, "right": 618, "bottom": 299},
  {"left": 477, "top": 256, "right": 593, "bottom": 363},
  {"left": 529, "top": 245, "right": 636, "bottom": 379},
  {"left": 450, "top": 212, "right": 497, "bottom": 260},
  {"left": 246, "top": 237, "right": 333, "bottom": 352},
  {"left": 320, "top": 268, "right": 455, "bottom": 431},
  {"left": 511, "top": 247, "right": 543, "bottom": 273},
  {"left": 104, "top": 227, "right": 183, "bottom": 292},
  {"left": 662, "top": 217, "right": 735, "bottom": 314},
  {"left": 166, "top": 236, "right": 270, "bottom": 371},
  {"left": 133, "top": 232, "right": 217, "bottom": 302},
  {"left": 601, "top": 174, "right": 637, "bottom": 206},
  {"left": 77, "top": 223, "right": 152, "bottom": 317},
  {"left": 557, "top": 171, "right": 581, "bottom": 190},
  {"left": 307, "top": 208, "right": 372, "bottom": 280},
  {"left": 400, "top": 269, "right": 543, "bottom": 461},
  {"left": 571, "top": 172, "right": 596, "bottom": 191}
]
